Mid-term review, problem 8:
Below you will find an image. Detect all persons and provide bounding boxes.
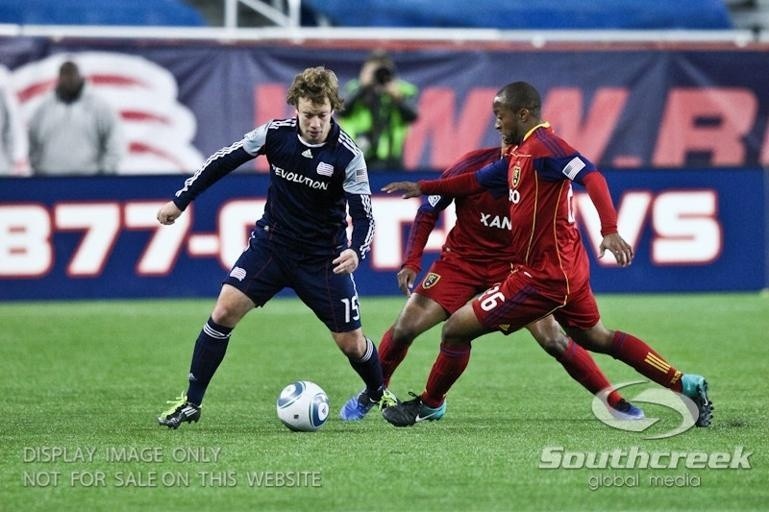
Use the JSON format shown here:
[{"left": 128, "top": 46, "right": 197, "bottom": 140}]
[
  {"left": 339, "top": 130, "right": 645, "bottom": 420},
  {"left": 26, "top": 60, "right": 128, "bottom": 177},
  {"left": 334, "top": 51, "right": 420, "bottom": 172},
  {"left": 380, "top": 79, "right": 715, "bottom": 428},
  {"left": 156, "top": 66, "right": 401, "bottom": 429}
]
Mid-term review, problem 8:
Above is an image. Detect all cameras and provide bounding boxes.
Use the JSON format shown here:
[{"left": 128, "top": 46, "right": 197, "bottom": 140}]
[{"left": 373, "top": 66, "right": 390, "bottom": 83}]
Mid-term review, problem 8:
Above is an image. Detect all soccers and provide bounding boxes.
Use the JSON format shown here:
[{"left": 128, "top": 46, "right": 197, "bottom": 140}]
[{"left": 277, "top": 381, "right": 329, "bottom": 431}]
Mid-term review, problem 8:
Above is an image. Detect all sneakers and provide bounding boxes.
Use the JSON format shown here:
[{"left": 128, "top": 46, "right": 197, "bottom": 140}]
[
  {"left": 369, "top": 384, "right": 402, "bottom": 412},
  {"left": 384, "top": 392, "right": 447, "bottom": 427},
  {"left": 339, "top": 386, "right": 375, "bottom": 424},
  {"left": 679, "top": 372, "right": 714, "bottom": 428},
  {"left": 157, "top": 390, "right": 203, "bottom": 430},
  {"left": 615, "top": 405, "right": 645, "bottom": 420}
]
